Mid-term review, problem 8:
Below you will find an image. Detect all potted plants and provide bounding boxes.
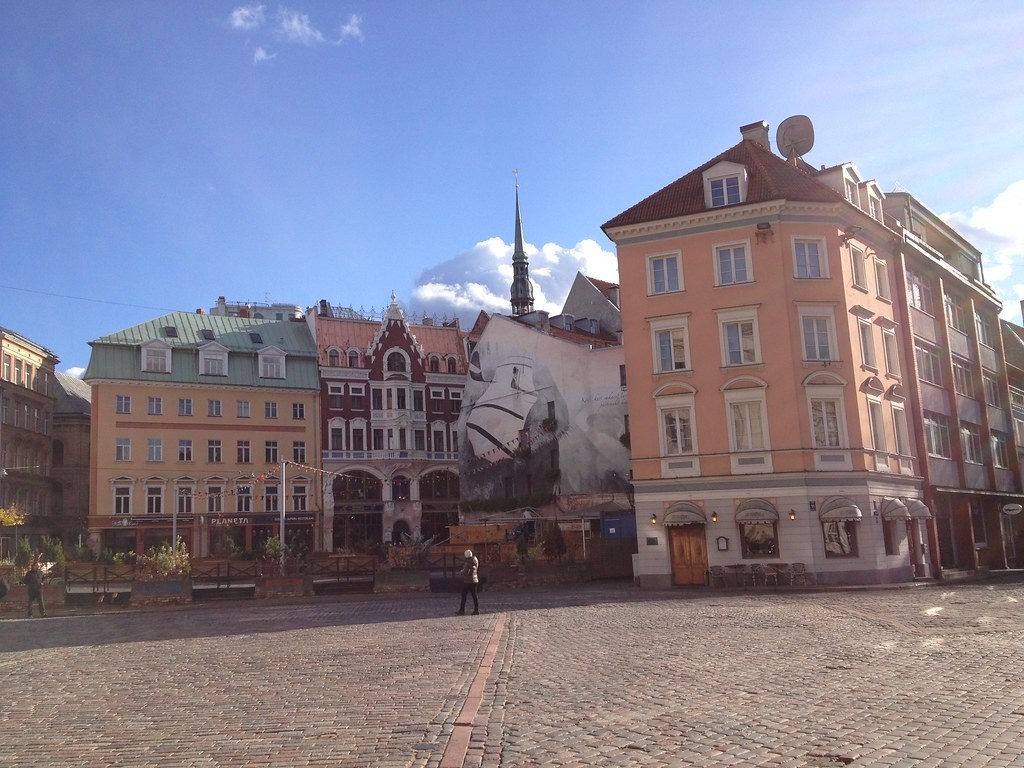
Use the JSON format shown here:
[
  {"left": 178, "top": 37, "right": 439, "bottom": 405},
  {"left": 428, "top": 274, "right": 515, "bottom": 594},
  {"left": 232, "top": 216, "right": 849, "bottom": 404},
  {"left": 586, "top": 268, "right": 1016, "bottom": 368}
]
[
  {"left": 135, "top": 534, "right": 192, "bottom": 596},
  {"left": 261, "top": 536, "right": 309, "bottom": 591},
  {"left": 386, "top": 532, "right": 441, "bottom": 594}
]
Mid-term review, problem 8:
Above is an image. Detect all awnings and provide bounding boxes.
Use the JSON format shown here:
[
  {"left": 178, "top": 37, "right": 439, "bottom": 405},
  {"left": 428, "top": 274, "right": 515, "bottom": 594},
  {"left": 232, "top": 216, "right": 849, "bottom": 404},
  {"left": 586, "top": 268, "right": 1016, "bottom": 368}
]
[
  {"left": 819, "top": 496, "right": 862, "bottom": 522},
  {"left": 736, "top": 499, "right": 778, "bottom": 524},
  {"left": 881, "top": 496, "right": 932, "bottom": 521},
  {"left": 661, "top": 501, "right": 707, "bottom": 526}
]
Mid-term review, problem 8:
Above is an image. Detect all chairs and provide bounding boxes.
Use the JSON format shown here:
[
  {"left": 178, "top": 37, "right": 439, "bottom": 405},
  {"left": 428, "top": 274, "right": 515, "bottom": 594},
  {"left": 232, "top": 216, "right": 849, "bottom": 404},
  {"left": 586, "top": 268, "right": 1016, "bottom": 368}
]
[{"left": 710, "top": 562, "right": 807, "bottom": 588}]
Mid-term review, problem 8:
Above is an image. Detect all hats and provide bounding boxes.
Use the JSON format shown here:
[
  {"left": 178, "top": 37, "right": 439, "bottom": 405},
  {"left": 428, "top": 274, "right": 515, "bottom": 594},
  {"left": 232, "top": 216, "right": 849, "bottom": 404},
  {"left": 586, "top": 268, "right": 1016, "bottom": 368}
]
[{"left": 465, "top": 550, "right": 472, "bottom": 558}]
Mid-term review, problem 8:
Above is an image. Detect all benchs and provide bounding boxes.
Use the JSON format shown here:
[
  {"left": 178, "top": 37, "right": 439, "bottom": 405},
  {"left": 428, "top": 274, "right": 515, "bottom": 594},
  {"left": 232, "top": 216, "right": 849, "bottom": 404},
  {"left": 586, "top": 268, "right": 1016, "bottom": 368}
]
[
  {"left": 310, "top": 559, "right": 376, "bottom": 596},
  {"left": 61, "top": 565, "right": 132, "bottom": 607},
  {"left": 190, "top": 562, "right": 258, "bottom": 602}
]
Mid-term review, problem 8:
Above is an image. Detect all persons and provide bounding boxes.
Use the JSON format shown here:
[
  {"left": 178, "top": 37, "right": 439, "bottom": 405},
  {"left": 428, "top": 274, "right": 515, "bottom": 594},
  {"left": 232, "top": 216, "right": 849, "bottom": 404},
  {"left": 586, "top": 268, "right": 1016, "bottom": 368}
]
[
  {"left": 455, "top": 550, "right": 480, "bottom": 615},
  {"left": 24, "top": 562, "right": 48, "bottom": 619}
]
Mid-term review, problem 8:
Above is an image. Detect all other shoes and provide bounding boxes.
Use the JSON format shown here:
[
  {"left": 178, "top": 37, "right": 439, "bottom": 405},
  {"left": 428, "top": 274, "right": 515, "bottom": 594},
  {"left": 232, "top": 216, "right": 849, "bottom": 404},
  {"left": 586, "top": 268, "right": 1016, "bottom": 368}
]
[
  {"left": 455, "top": 611, "right": 464, "bottom": 615},
  {"left": 28, "top": 615, "right": 34, "bottom": 619},
  {"left": 471, "top": 611, "right": 480, "bottom": 615},
  {"left": 41, "top": 613, "right": 47, "bottom": 618}
]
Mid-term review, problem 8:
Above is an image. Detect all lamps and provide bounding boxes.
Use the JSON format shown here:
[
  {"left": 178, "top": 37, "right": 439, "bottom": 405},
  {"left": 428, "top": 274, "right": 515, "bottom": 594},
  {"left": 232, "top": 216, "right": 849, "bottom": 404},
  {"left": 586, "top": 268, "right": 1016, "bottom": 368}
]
[
  {"left": 885, "top": 238, "right": 905, "bottom": 257},
  {"left": 711, "top": 511, "right": 718, "bottom": 522},
  {"left": 650, "top": 514, "right": 657, "bottom": 524},
  {"left": 789, "top": 509, "right": 795, "bottom": 520},
  {"left": 841, "top": 225, "right": 862, "bottom": 241},
  {"left": 756, "top": 223, "right": 774, "bottom": 242}
]
